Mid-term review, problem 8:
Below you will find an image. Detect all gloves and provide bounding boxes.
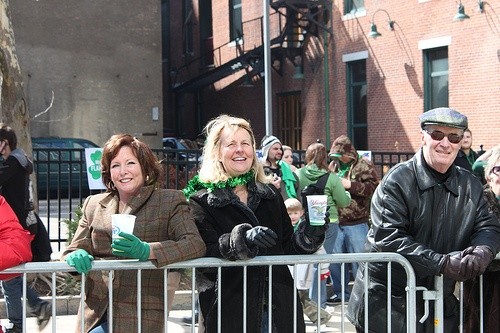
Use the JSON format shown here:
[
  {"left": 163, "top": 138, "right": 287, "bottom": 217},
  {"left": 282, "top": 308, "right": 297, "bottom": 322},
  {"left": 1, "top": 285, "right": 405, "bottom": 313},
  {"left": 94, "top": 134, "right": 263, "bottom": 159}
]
[
  {"left": 458, "top": 245, "right": 494, "bottom": 281},
  {"left": 111, "top": 232, "right": 150, "bottom": 262},
  {"left": 304, "top": 205, "right": 331, "bottom": 239},
  {"left": 245, "top": 226, "right": 278, "bottom": 250},
  {"left": 65, "top": 248, "right": 94, "bottom": 275},
  {"left": 442, "top": 246, "right": 475, "bottom": 281}
]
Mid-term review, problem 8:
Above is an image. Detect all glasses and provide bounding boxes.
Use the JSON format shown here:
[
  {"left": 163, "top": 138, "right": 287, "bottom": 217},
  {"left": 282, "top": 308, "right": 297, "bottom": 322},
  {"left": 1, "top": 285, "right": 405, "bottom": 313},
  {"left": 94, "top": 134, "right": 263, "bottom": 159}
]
[
  {"left": 489, "top": 166, "right": 500, "bottom": 177},
  {"left": 423, "top": 129, "right": 464, "bottom": 143}
]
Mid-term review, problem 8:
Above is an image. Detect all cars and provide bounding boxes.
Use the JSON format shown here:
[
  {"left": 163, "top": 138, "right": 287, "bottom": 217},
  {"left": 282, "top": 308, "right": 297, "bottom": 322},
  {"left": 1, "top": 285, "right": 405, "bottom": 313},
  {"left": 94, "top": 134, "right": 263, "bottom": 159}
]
[{"left": 31, "top": 137, "right": 102, "bottom": 200}]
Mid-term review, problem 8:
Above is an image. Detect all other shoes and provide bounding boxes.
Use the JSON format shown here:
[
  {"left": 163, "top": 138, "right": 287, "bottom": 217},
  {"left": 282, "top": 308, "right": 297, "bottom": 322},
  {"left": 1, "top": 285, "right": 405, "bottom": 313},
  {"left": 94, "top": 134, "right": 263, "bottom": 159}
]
[
  {"left": 314, "top": 313, "right": 332, "bottom": 331},
  {"left": 36, "top": 302, "right": 52, "bottom": 331},
  {"left": 4, "top": 328, "right": 22, "bottom": 333},
  {"left": 326, "top": 294, "right": 350, "bottom": 303}
]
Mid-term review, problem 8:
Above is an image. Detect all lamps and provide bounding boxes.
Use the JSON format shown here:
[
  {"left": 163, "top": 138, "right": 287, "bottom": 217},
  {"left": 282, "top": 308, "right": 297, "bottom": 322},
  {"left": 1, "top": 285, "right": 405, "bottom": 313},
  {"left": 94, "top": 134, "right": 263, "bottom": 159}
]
[
  {"left": 369, "top": 8, "right": 395, "bottom": 38},
  {"left": 454, "top": 0, "right": 485, "bottom": 21}
]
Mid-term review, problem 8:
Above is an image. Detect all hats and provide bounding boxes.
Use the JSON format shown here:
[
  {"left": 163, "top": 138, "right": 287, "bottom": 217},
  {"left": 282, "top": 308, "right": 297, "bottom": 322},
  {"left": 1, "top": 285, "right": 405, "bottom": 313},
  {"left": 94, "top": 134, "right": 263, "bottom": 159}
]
[
  {"left": 260, "top": 135, "right": 285, "bottom": 169},
  {"left": 420, "top": 107, "right": 468, "bottom": 131}
]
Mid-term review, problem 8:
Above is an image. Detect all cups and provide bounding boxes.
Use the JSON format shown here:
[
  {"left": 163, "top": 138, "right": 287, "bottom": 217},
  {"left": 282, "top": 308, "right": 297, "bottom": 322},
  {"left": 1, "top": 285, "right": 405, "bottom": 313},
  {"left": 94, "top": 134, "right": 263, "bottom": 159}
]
[
  {"left": 306, "top": 195, "right": 328, "bottom": 226},
  {"left": 111, "top": 214, "right": 136, "bottom": 252}
]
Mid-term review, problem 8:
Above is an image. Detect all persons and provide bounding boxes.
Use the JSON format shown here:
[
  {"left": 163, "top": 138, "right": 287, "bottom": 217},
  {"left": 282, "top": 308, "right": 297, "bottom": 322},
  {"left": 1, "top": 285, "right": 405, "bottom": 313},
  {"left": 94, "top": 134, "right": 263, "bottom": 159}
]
[
  {"left": 455, "top": 129, "right": 487, "bottom": 185},
  {"left": 283, "top": 199, "right": 335, "bottom": 331},
  {"left": 344, "top": 107, "right": 500, "bottom": 333},
  {"left": 472, "top": 148, "right": 495, "bottom": 186},
  {"left": 0, "top": 196, "right": 35, "bottom": 333},
  {"left": 189, "top": 115, "right": 330, "bottom": 333},
  {"left": 279, "top": 144, "right": 298, "bottom": 172},
  {"left": 460, "top": 145, "right": 500, "bottom": 333},
  {"left": 294, "top": 143, "right": 351, "bottom": 308},
  {"left": 0, "top": 122, "right": 52, "bottom": 333},
  {"left": 59, "top": 135, "right": 206, "bottom": 333},
  {"left": 257, "top": 135, "right": 299, "bottom": 279},
  {"left": 328, "top": 136, "right": 379, "bottom": 304}
]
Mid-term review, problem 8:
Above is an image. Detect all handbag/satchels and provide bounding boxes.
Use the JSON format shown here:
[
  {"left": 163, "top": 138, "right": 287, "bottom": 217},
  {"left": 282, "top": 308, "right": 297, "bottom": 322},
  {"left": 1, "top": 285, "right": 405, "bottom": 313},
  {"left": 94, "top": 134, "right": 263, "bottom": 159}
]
[
  {"left": 25, "top": 210, "right": 53, "bottom": 263},
  {"left": 301, "top": 171, "right": 330, "bottom": 205}
]
